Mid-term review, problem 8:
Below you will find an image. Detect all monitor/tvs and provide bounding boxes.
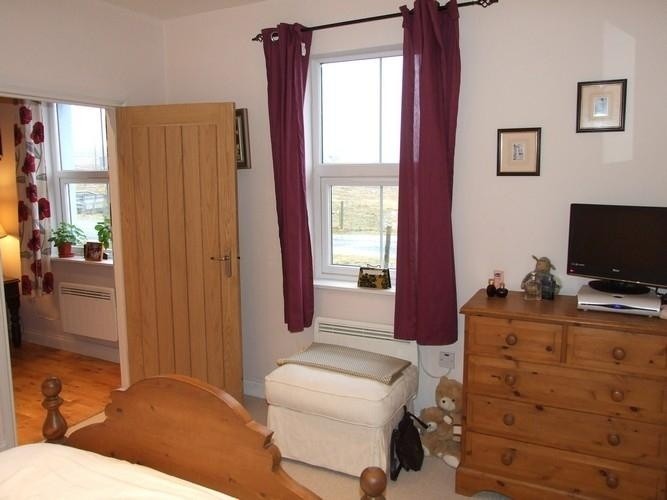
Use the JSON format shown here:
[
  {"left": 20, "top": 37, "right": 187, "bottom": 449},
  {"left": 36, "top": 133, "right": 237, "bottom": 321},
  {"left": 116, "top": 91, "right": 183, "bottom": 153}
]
[{"left": 566, "top": 202, "right": 667, "bottom": 296}]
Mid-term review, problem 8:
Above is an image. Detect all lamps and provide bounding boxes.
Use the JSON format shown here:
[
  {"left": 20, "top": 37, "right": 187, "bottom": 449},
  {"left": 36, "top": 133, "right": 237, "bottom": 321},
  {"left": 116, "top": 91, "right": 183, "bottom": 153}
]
[{"left": 0, "top": 223, "right": 8, "bottom": 239}]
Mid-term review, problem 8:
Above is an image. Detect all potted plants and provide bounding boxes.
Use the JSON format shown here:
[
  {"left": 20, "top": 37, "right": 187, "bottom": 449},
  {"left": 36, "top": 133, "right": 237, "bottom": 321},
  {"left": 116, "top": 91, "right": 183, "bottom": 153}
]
[{"left": 48, "top": 216, "right": 88, "bottom": 258}]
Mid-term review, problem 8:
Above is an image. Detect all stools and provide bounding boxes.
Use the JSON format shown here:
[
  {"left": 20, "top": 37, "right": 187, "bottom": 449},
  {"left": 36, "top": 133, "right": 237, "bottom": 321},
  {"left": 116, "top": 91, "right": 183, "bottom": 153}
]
[{"left": 264, "top": 342, "right": 419, "bottom": 479}]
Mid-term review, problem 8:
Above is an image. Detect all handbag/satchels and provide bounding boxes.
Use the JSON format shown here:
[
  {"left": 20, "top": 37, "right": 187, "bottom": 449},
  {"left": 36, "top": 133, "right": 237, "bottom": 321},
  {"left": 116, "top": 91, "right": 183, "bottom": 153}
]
[{"left": 391, "top": 405, "right": 428, "bottom": 481}]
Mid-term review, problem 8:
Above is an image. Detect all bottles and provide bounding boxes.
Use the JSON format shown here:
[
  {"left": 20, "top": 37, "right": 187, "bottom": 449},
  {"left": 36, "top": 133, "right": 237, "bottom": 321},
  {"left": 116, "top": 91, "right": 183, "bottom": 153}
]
[
  {"left": 487, "top": 278, "right": 508, "bottom": 298},
  {"left": 523, "top": 273, "right": 541, "bottom": 303}
]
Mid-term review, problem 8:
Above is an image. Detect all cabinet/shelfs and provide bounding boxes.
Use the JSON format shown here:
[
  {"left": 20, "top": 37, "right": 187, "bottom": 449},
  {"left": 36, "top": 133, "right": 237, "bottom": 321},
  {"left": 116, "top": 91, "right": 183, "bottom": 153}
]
[{"left": 457, "top": 290, "right": 667, "bottom": 500}]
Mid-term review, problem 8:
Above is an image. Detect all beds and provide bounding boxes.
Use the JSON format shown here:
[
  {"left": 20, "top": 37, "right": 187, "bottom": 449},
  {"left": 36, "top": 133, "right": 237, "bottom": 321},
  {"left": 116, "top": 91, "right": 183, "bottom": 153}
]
[{"left": 1, "top": 373, "right": 386, "bottom": 500}]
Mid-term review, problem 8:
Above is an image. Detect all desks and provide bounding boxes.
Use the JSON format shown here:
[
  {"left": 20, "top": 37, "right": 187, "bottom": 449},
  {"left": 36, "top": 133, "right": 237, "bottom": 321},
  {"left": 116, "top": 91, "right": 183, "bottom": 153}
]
[{"left": 4, "top": 277, "right": 21, "bottom": 348}]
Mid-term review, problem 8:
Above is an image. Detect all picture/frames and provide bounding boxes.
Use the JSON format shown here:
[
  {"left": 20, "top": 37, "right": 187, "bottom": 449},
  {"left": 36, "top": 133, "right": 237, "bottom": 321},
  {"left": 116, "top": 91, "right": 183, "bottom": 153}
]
[
  {"left": 576, "top": 79, "right": 627, "bottom": 133},
  {"left": 235, "top": 108, "right": 252, "bottom": 169},
  {"left": 496, "top": 127, "right": 541, "bottom": 176}
]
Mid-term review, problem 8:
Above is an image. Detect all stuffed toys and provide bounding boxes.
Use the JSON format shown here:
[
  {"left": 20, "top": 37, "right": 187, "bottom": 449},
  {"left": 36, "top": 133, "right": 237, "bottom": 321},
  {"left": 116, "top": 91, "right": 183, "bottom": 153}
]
[
  {"left": 412, "top": 406, "right": 453, "bottom": 459},
  {"left": 434, "top": 374, "right": 463, "bottom": 468}
]
